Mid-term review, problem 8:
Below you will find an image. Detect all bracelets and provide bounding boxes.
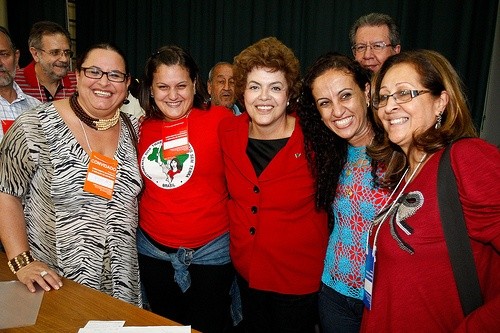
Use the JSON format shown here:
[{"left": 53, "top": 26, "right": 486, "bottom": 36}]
[{"left": 7, "top": 250, "right": 36, "bottom": 274}]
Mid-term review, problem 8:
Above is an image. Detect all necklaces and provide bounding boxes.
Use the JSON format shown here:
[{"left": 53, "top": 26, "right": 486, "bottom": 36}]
[
  {"left": 36, "top": 72, "right": 66, "bottom": 104},
  {"left": 345, "top": 133, "right": 376, "bottom": 173},
  {"left": 69, "top": 92, "right": 121, "bottom": 131}
]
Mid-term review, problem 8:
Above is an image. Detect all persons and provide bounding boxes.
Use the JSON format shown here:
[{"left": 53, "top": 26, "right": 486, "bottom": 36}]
[
  {"left": 361, "top": 49, "right": 500, "bottom": 333},
  {"left": 200, "top": 62, "right": 242, "bottom": 116},
  {"left": 296, "top": 56, "right": 396, "bottom": 333},
  {"left": 138, "top": 36, "right": 332, "bottom": 333},
  {"left": 15, "top": 21, "right": 79, "bottom": 104},
  {"left": 135, "top": 45, "right": 244, "bottom": 333},
  {"left": 350, "top": 13, "right": 401, "bottom": 77},
  {"left": 0, "top": 41, "right": 144, "bottom": 311},
  {"left": 0, "top": 25, "right": 45, "bottom": 149}
]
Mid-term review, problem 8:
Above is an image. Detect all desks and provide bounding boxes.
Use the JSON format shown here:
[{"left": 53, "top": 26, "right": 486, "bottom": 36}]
[{"left": 0, "top": 251, "right": 204, "bottom": 333}]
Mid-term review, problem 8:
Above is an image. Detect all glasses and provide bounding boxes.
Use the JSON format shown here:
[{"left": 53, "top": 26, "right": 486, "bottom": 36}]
[
  {"left": 36, "top": 48, "right": 74, "bottom": 58},
  {"left": 79, "top": 67, "right": 128, "bottom": 83},
  {"left": 352, "top": 42, "right": 393, "bottom": 52},
  {"left": 372, "top": 90, "right": 431, "bottom": 108}
]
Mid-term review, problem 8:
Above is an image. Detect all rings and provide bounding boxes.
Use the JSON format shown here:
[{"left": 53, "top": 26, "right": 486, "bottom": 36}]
[{"left": 40, "top": 270, "right": 48, "bottom": 278}]
